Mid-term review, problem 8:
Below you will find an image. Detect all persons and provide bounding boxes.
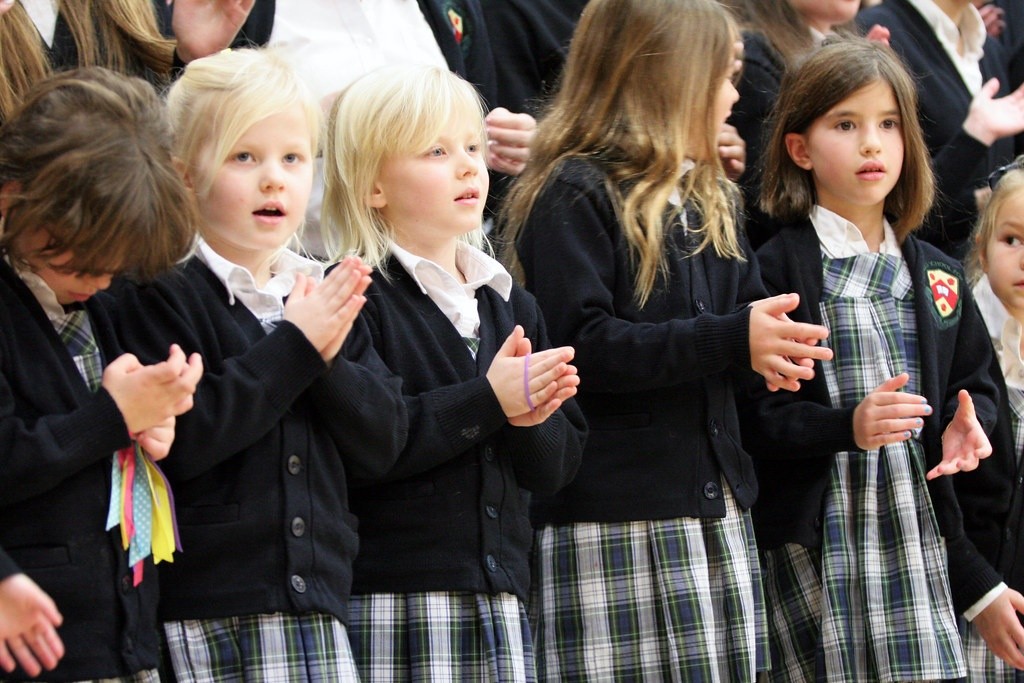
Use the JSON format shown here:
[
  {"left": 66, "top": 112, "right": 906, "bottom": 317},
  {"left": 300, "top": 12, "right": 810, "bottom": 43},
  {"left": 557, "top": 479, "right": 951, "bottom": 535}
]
[
  {"left": 933, "top": 154, "right": 1024, "bottom": 683},
  {"left": 92, "top": 38, "right": 374, "bottom": 683},
  {"left": 0, "top": 0, "right": 1024, "bottom": 290},
  {"left": 757, "top": 35, "right": 1001, "bottom": 683},
  {"left": 0, "top": 549, "right": 64, "bottom": 677},
  {"left": 0, "top": 67, "right": 206, "bottom": 683},
  {"left": 500, "top": 1, "right": 834, "bottom": 683},
  {"left": 300, "top": 65, "right": 591, "bottom": 682}
]
